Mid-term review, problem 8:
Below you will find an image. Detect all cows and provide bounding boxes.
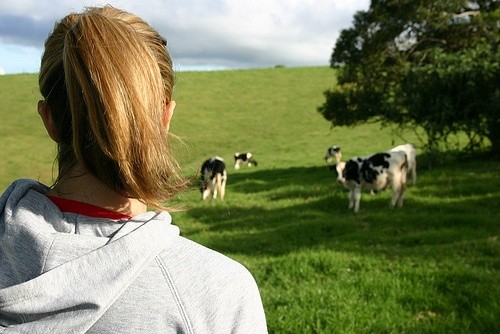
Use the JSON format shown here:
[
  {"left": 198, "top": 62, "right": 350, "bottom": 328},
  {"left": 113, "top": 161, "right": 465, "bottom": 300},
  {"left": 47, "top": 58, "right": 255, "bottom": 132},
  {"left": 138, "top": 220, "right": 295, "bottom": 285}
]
[
  {"left": 330, "top": 149, "right": 409, "bottom": 213},
  {"left": 234, "top": 151, "right": 258, "bottom": 171},
  {"left": 369, "top": 143, "right": 417, "bottom": 196},
  {"left": 197, "top": 156, "right": 228, "bottom": 201},
  {"left": 324, "top": 145, "right": 342, "bottom": 164}
]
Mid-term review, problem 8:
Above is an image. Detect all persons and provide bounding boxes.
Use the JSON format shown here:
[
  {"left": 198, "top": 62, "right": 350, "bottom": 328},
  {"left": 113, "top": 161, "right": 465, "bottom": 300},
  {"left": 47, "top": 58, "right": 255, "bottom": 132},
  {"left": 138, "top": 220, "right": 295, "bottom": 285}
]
[{"left": 0, "top": 4, "right": 271, "bottom": 334}]
